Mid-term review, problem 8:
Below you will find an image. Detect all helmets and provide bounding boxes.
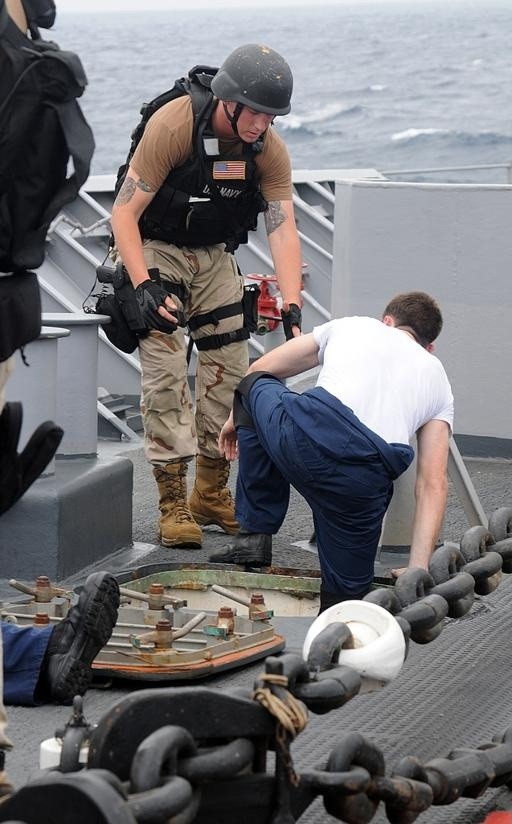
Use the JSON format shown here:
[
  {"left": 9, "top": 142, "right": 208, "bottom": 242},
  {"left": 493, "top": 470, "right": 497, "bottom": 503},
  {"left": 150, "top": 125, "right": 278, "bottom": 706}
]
[{"left": 211, "top": 43, "right": 295, "bottom": 117}]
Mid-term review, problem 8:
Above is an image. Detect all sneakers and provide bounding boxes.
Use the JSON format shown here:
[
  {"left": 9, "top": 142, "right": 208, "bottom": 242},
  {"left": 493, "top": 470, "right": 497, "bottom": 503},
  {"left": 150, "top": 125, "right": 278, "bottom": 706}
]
[
  {"left": 39, "top": 570, "right": 120, "bottom": 706},
  {"left": 207, "top": 532, "right": 273, "bottom": 568}
]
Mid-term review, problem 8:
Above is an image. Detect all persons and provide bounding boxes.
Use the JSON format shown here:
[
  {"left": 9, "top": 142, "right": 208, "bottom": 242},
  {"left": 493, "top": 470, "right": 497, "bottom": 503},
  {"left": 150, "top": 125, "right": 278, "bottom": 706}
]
[
  {"left": 208, "top": 292, "right": 451, "bottom": 621},
  {"left": 0, "top": 0, "right": 95, "bottom": 523},
  {"left": 0, "top": 570, "right": 121, "bottom": 708},
  {"left": 114, "top": 44, "right": 312, "bottom": 544}
]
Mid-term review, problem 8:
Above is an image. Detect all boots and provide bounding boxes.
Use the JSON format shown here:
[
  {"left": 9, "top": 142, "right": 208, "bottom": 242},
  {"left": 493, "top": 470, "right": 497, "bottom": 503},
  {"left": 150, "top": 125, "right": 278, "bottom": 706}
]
[
  {"left": 152, "top": 457, "right": 203, "bottom": 551},
  {"left": 189, "top": 453, "right": 241, "bottom": 536}
]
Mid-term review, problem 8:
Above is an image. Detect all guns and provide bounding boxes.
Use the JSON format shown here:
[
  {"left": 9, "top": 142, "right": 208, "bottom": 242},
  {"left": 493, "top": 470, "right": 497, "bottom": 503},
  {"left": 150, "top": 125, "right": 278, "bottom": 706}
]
[{"left": 98, "top": 264, "right": 147, "bottom": 333}]
